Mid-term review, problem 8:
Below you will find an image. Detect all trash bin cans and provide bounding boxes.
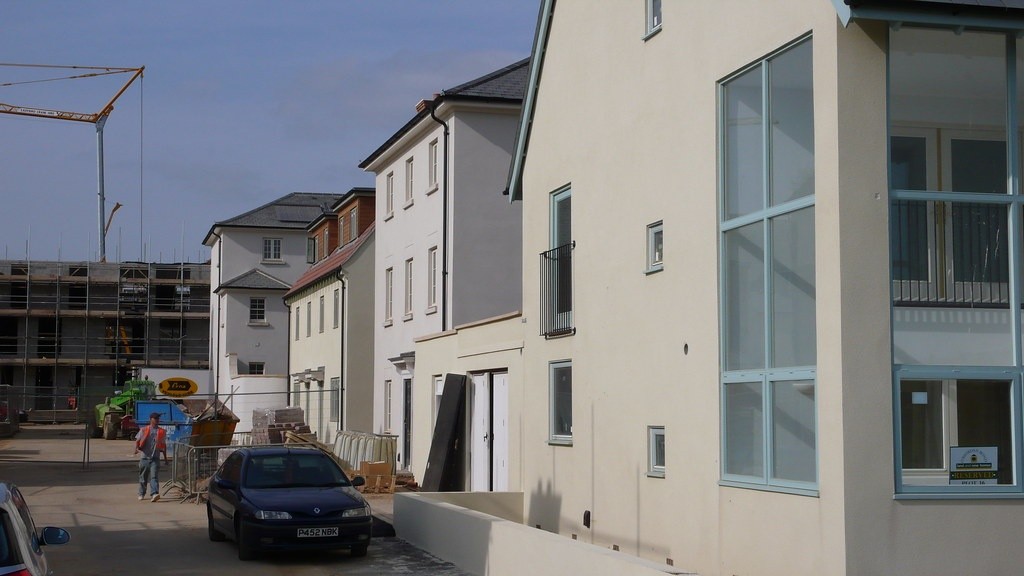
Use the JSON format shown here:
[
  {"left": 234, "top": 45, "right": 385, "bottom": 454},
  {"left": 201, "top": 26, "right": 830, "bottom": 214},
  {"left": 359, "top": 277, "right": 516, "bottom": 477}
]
[{"left": 133, "top": 397, "right": 241, "bottom": 458}]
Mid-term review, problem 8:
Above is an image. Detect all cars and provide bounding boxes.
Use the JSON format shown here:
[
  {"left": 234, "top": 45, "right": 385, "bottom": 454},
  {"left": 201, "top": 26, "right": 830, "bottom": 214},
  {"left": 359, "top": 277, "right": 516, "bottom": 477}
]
[
  {"left": 206, "top": 446, "right": 374, "bottom": 560},
  {"left": 0, "top": 480, "right": 72, "bottom": 576}
]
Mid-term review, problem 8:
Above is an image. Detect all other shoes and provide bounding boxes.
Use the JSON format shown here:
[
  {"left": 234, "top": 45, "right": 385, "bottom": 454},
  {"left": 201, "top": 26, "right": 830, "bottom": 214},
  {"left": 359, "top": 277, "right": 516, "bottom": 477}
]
[
  {"left": 151, "top": 494, "right": 160, "bottom": 502},
  {"left": 138, "top": 495, "right": 144, "bottom": 500}
]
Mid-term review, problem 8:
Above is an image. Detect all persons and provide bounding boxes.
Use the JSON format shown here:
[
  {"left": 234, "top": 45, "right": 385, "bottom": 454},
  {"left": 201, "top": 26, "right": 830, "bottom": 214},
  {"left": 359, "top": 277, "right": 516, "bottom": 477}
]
[{"left": 134, "top": 413, "right": 168, "bottom": 502}]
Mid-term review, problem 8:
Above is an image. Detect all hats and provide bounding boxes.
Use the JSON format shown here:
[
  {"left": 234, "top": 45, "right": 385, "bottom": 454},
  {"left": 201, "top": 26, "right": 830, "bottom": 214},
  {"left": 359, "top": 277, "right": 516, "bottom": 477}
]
[{"left": 150, "top": 413, "right": 162, "bottom": 419}]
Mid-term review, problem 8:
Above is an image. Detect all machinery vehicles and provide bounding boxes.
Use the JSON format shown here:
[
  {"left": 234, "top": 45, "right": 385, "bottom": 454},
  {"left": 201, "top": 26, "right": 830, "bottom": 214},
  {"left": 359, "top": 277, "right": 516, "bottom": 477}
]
[{"left": 88, "top": 380, "right": 157, "bottom": 441}]
